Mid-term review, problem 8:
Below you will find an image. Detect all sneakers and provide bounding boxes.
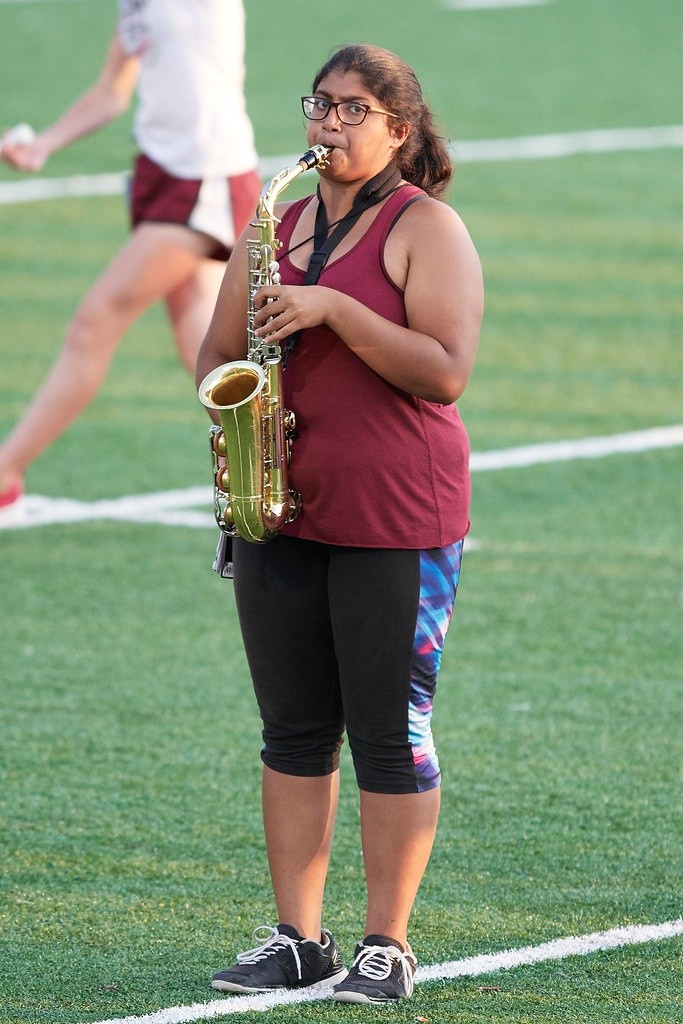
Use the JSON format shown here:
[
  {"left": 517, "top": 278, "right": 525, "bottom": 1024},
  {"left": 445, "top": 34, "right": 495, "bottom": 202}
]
[
  {"left": 332, "top": 934, "right": 417, "bottom": 1005},
  {"left": 211, "top": 924, "right": 349, "bottom": 993}
]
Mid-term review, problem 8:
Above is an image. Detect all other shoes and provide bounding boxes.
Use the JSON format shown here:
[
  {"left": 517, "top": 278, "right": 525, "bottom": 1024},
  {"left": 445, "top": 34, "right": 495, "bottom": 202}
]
[{"left": 0, "top": 474, "right": 22, "bottom": 507}]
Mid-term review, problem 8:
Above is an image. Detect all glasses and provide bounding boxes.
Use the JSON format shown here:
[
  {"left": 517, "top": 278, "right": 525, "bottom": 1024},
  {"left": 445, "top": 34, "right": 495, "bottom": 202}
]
[{"left": 301, "top": 96, "right": 400, "bottom": 125}]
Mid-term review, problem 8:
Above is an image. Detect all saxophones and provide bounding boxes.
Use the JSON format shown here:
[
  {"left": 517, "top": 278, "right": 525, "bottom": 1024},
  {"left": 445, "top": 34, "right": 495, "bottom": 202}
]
[{"left": 196, "top": 142, "right": 339, "bottom": 546}]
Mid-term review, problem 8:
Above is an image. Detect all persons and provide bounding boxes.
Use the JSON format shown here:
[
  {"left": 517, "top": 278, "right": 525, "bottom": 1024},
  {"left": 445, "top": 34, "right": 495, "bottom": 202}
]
[
  {"left": 195, "top": 45, "right": 486, "bottom": 1005},
  {"left": 0, "top": 0, "right": 264, "bottom": 511}
]
